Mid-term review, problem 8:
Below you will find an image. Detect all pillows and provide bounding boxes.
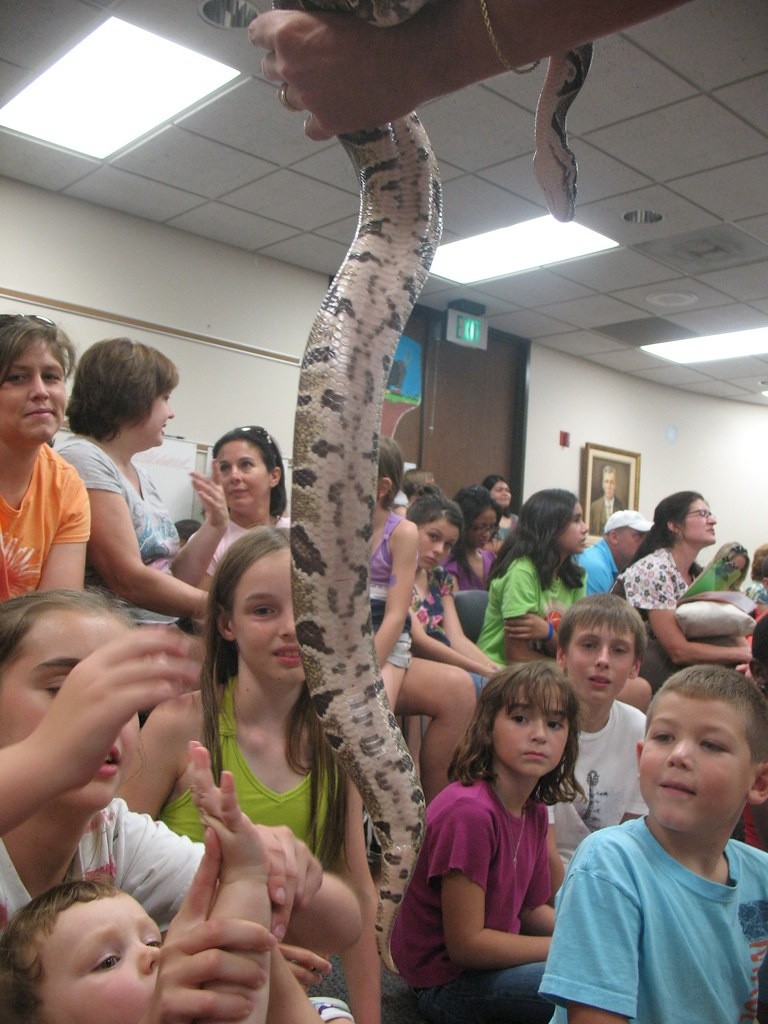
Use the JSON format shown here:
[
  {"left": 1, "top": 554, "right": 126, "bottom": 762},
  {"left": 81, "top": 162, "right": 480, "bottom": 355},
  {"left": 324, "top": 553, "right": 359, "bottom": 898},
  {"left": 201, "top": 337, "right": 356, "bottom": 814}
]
[{"left": 675, "top": 600, "right": 758, "bottom": 640}]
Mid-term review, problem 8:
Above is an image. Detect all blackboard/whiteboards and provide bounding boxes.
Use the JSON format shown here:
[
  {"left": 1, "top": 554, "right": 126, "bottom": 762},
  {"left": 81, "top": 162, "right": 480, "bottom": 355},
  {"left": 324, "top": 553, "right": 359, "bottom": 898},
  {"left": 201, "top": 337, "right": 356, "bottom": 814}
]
[{"left": 53, "top": 427, "right": 198, "bottom": 524}]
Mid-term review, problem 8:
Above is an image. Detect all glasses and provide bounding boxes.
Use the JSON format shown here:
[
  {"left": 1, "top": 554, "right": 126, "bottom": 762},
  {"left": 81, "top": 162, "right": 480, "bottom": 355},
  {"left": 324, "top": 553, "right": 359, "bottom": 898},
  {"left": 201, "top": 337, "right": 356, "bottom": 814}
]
[
  {"left": 0, "top": 315, "right": 55, "bottom": 328},
  {"left": 684, "top": 509, "right": 713, "bottom": 519},
  {"left": 239, "top": 426, "right": 275, "bottom": 460}
]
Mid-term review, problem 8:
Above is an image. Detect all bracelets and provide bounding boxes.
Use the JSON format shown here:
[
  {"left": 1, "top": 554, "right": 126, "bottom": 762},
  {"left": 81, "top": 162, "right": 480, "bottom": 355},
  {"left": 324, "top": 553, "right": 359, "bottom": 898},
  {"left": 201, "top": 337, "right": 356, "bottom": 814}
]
[{"left": 477, "top": 0, "right": 540, "bottom": 75}]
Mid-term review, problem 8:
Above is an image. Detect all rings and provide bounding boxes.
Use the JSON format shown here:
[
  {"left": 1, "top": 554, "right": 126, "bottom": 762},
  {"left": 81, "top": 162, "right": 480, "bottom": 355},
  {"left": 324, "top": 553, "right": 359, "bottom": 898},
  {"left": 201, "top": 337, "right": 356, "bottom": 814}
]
[{"left": 278, "top": 84, "right": 302, "bottom": 114}]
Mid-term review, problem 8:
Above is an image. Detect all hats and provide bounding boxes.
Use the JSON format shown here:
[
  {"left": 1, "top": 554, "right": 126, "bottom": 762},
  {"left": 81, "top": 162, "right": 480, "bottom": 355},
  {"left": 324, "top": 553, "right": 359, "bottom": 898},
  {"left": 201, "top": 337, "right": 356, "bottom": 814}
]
[{"left": 604, "top": 510, "right": 652, "bottom": 534}]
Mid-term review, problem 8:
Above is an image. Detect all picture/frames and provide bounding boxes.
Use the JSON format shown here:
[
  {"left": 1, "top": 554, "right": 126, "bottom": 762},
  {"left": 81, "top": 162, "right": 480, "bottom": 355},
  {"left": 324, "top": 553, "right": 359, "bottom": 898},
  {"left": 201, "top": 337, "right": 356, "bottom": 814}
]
[{"left": 580, "top": 444, "right": 643, "bottom": 541}]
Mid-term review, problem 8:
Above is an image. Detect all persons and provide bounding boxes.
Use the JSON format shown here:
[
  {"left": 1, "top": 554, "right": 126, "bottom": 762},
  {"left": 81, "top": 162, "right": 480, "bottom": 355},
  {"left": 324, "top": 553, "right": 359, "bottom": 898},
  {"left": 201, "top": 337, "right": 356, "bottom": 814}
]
[
  {"left": 0, "top": 592, "right": 355, "bottom": 1024},
  {"left": 537, "top": 667, "right": 768, "bottom": 1024},
  {"left": 116, "top": 527, "right": 386, "bottom": 1023},
  {"left": 389, "top": 661, "right": 552, "bottom": 1024},
  {"left": 542, "top": 591, "right": 652, "bottom": 880},
  {"left": 373, "top": 436, "right": 586, "bottom": 814},
  {"left": 0, "top": 315, "right": 92, "bottom": 601},
  {"left": 588, "top": 492, "right": 768, "bottom": 851},
  {"left": 591, "top": 465, "right": 626, "bottom": 537},
  {"left": 193, "top": 426, "right": 297, "bottom": 634},
  {"left": 56, "top": 337, "right": 226, "bottom": 625},
  {"left": 248, "top": 0, "right": 698, "bottom": 141}
]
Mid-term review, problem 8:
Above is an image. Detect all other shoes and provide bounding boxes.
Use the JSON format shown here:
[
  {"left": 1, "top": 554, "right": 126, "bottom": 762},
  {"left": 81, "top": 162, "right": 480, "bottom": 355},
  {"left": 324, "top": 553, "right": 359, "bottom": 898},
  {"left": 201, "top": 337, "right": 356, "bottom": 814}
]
[{"left": 312, "top": 997, "right": 354, "bottom": 1023}]
